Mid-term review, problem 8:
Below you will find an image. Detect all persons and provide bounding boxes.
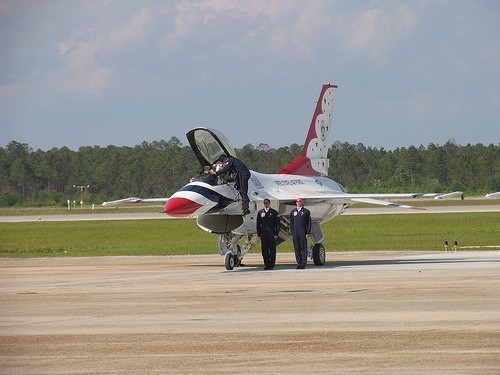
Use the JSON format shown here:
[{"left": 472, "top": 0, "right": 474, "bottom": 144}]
[
  {"left": 185, "top": 154, "right": 251, "bottom": 213},
  {"left": 257, "top": 199, "right": 280, "bottom": 270},
  {"left": 290, "top": 198, "right": 311, "bottom": 269}
]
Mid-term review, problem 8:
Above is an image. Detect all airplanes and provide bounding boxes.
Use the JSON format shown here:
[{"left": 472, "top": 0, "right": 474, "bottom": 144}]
[{"left": 101, "top": 82, "right": 464, "bottom": 270}]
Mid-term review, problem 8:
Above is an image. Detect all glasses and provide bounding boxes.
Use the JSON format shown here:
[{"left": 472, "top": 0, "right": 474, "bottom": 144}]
[
  {"left": 296, "top": 201, "right": 302, "bottom": 202},
  {"left": 263, "top": 202, "right": 269, "bottom": 204}
]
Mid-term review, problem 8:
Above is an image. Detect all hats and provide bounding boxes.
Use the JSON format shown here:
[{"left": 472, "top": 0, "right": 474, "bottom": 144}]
[{"left": 220, "top": 154, "right": 225, "bottom": 162}]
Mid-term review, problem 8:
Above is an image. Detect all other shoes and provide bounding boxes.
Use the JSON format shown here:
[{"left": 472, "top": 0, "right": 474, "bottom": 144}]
[
  {"left": 264, "top": 267, "right": 273, "bottom": 270},
  {"left": 243, "top": 210, "right": 250, "bottom": 214},
  {"left": 297, "top": 265, "right": 301, "bottom": 269},
  {"left": 301, "top": 266, "right": 305, "bottom": 269}
]
